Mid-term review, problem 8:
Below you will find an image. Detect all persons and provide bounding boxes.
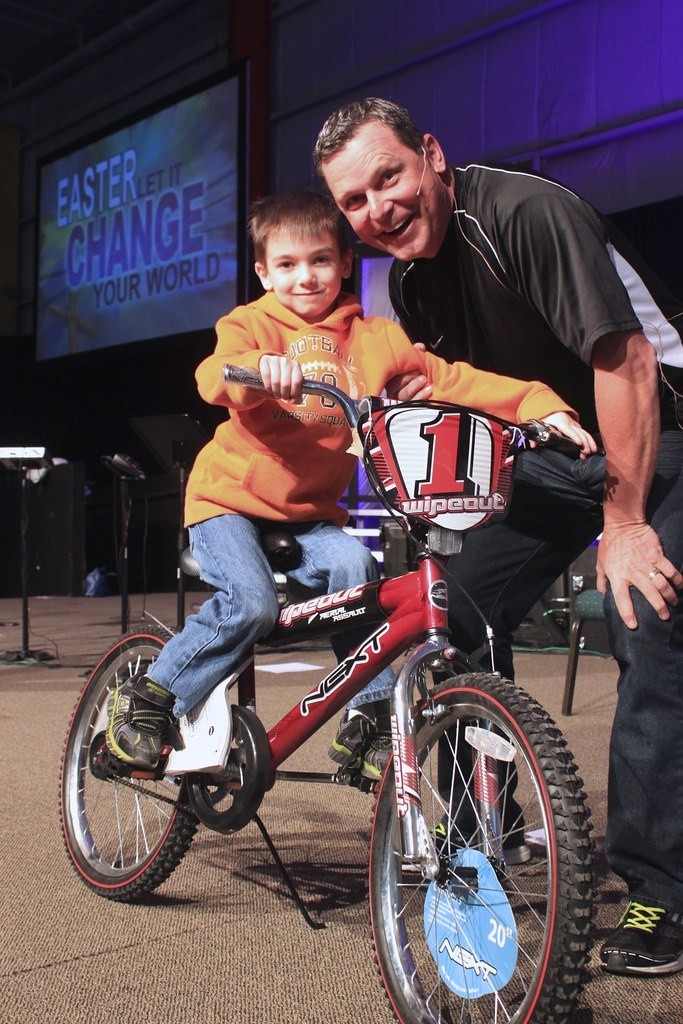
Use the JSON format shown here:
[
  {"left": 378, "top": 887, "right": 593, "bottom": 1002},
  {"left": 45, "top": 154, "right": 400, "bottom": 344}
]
[
  {"left": 108, "top": 187, "right": 598, "bottom": 782},
  {"left": 311, "top": 95, "right": 683, "bottom": 978}
]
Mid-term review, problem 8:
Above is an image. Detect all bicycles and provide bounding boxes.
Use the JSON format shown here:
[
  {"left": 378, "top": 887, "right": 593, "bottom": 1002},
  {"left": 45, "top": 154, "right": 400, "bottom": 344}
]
[{"left": 57, "top": 363, "right": 601, "bottom": 1023}]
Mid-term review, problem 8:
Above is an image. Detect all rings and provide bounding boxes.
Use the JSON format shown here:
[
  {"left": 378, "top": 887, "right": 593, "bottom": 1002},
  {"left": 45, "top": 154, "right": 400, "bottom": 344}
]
[{"left": 648, "top": 568, "right": 660, "bottom": 580}]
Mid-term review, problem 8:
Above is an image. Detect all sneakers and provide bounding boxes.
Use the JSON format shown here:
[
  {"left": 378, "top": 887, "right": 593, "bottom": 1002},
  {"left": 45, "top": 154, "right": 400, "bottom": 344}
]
[
  {"left": 399, "top": 817, "right": 530, "bottom": 872},
  {"left": 328, "top": 708, "right": 395, "bottom": 781},
  {"left": 599, "top": 899, "right": 683, "bottom": 977},
  {"left": 105, "top": 662, "right": 182, "bottom": 770}
]
